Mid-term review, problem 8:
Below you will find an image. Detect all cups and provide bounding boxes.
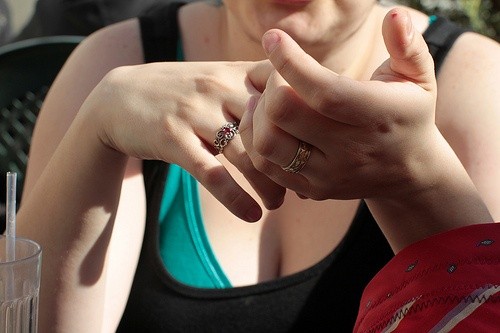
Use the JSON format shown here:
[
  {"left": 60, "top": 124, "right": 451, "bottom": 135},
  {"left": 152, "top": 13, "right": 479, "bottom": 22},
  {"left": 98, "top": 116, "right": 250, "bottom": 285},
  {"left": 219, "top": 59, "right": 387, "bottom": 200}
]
[{"left": 0, "top": 236, "right": 42, "bottom": 333}]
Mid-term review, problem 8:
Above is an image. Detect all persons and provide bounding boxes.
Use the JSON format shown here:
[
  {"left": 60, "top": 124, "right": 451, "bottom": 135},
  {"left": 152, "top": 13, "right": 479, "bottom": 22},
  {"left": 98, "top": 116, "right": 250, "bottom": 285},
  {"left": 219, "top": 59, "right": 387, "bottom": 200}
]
[{"left": 0, "top": 0, "right": 499, "bottom": 333}]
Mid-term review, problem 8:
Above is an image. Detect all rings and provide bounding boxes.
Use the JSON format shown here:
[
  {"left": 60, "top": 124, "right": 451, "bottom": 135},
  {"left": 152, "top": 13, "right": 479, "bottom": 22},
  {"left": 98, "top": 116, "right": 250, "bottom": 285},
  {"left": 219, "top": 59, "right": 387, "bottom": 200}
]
[
  {"left": 210, "top": 121, "right": 240, "bottom": 154},
  {"left": 281, "top": 141, "right": 312, "bottom": 174}
]
